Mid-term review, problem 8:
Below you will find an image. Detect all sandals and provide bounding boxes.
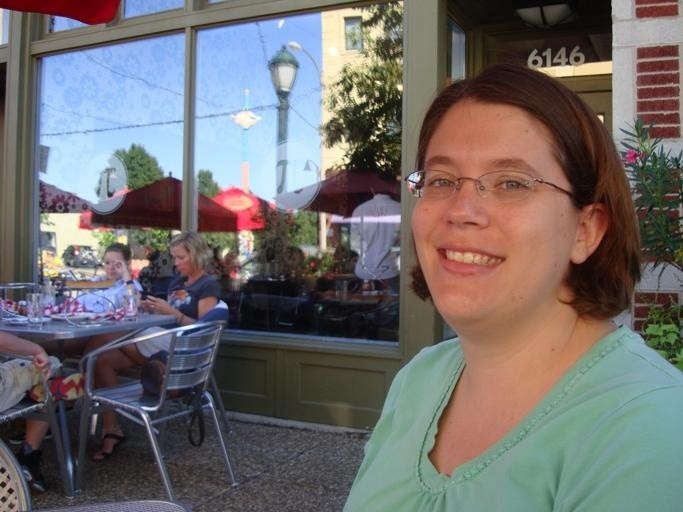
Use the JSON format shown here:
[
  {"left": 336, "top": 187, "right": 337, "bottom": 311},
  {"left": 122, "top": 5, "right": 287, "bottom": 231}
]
[{"left": 92, "top": 434, "right": 128, "bottom": 462}]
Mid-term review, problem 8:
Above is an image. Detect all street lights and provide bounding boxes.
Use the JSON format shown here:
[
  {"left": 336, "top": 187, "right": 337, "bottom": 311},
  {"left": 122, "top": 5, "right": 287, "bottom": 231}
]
[
  {"left": 265, "top": 42, "right": 299, "bottom": 241},
  {"left": 285, "top": 37, "right": 321, "bottom": 76}
]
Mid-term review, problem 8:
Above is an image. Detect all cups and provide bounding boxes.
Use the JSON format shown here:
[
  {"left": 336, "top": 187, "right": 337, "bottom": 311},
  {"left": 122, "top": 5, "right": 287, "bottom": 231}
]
[
  {"left": 26, "top": 282, "right": 58, "bottom": 328},
  {"left": 105, "top": 279, "right": 138, "bottom": 322},
  {"left": 334, "top": 280, "right": 348, "bottom": 298}
]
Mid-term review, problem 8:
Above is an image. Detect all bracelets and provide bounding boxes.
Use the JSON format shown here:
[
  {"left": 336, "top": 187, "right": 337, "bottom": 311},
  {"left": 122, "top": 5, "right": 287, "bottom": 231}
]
[
  {"left": 124, "top": 279, "right": 134, "bottom": 286},
  {"left": 179, "top": 314, "right": 185, "bottom": 325}
]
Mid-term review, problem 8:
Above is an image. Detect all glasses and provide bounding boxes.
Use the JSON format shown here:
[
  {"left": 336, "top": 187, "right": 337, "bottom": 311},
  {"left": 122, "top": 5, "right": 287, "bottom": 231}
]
[{"left": 404, "top": 170, "right": 578, "bottom": 203}]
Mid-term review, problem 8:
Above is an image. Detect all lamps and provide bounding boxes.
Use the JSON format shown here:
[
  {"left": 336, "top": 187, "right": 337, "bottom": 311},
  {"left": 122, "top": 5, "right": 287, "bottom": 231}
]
[{"left": 514, "top": 3, "right": 576, "bottom": 30}]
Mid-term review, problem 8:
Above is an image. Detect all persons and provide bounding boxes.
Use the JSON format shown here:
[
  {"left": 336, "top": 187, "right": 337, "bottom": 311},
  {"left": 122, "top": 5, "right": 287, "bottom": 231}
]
[
  {"left": 133, "top": 233, "right": 399, "bottom": 339},
  {"left": 0, "top": 329, "right": 62, "bottom": 494},
  {"left": 331, "top": 62, "right": 683, "bottom": 511},
  {"left": 348, "top": 183, "right": 399, "bottom": 291},
  {"left": 65, "top": 242, "right": 144, "bottom": 357},
  {"left": 92, "top": 229, "right": 222, "bottom": 464}
]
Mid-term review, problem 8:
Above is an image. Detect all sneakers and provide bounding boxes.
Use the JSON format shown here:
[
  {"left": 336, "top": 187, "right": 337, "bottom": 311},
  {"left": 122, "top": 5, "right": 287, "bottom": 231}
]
[
  {"left": 30, "top": 373, "right": 85, "bottom": 402},
  {"left": 9, "top": 425, "right": 52, "bottom": 445},
  {"left": 15, "top": 446, "right": 46, "bottom": 492}
]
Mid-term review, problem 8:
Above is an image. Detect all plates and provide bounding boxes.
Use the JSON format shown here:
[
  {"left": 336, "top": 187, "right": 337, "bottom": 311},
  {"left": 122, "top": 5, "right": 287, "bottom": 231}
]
[{"left": 4, "top": 311, "right": 93, "bottom": 326}]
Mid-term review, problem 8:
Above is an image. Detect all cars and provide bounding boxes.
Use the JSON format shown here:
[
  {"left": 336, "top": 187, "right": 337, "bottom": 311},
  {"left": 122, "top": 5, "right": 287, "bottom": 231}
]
[
  {"left": 61, "top": 244, "right": 95, "bottom": 268},
  {"left": 222, "top": 265, "right": 399, "bottom": 340}
]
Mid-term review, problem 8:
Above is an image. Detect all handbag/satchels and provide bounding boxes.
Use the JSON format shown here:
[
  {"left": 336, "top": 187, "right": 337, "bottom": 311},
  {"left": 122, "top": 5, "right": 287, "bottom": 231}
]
[{"left": 142, "top": 348, "right": 210, "bottom": 399}]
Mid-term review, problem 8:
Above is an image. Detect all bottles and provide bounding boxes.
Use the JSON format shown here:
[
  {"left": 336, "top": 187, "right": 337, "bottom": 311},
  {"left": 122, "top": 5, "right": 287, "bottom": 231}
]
[{"left": 361, "top": 279, "right": 371, "bottom": 290}]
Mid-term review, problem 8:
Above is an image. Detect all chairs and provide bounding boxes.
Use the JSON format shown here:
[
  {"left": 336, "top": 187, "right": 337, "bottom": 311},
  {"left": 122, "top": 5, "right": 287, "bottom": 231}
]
[{"left": 221, "top": 276, "right": 400, "bottom": 340}]
